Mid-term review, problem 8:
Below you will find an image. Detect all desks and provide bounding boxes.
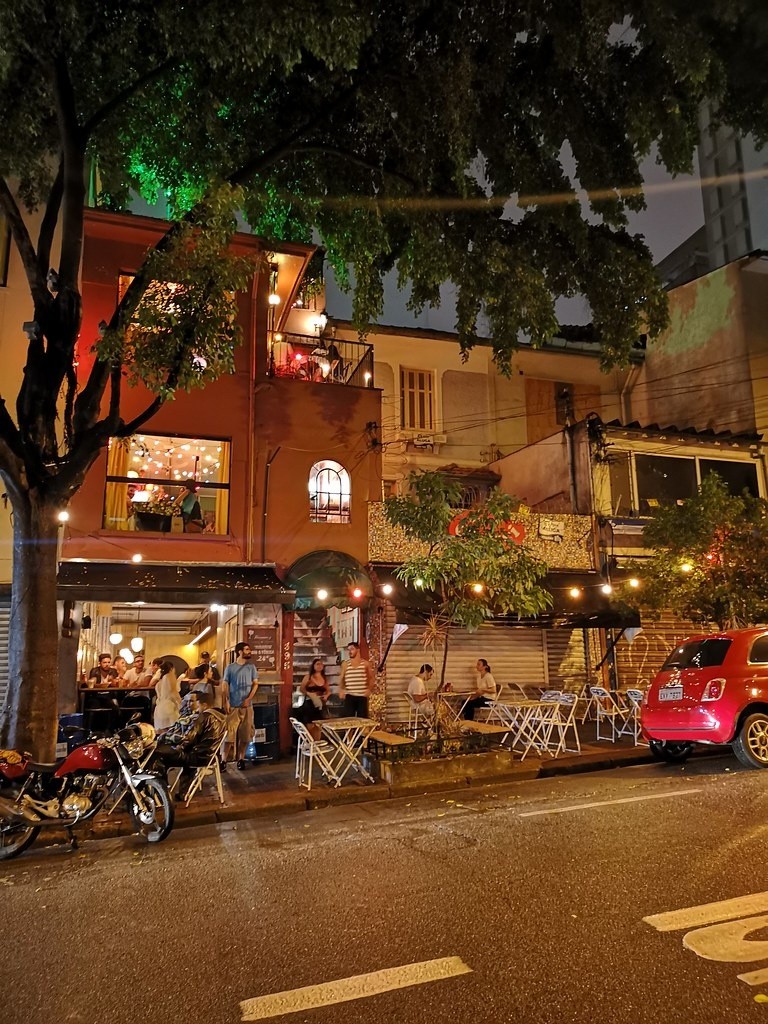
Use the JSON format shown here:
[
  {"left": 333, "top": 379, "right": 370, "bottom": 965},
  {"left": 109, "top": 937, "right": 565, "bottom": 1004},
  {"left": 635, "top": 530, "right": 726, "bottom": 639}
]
[
  {"left": 615, "top": 683, "right": 651, "bottom": 746},
  {"left": 433, "top": 690, "right": 478, "bottom": 722},
  {"left": 312, "top": 717, "right": 379, "bottom": 789},
  {"left": 483, "top": 700, "right": 560, "bottom": 761},
  {"left": 524, "top": 682, "right": 569, "bottom": 724},
  {"left": 79, "top": 685, "right": 155, "bottom": 723}
]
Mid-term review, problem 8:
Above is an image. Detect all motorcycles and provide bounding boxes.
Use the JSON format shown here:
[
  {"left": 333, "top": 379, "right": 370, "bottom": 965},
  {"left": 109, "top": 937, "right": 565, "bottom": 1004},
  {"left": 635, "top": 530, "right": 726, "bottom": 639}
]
[{"left": 0, "top": 712, "right": 176, "bottom": 862}]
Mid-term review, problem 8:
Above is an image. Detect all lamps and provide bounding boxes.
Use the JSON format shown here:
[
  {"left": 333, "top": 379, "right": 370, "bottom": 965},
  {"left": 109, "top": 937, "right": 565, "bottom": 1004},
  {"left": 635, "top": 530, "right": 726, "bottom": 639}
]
[
  {"left": 108, "top": 605, "right": 145, "bottom": 666},
  {"left": 596, "top": 518, "right": 617, "bottom": 575}
]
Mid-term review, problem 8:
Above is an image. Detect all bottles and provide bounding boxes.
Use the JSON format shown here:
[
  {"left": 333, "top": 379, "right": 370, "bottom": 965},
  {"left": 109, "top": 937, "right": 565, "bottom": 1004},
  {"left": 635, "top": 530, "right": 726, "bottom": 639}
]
[
  {"left": 245, "top": 742, "right": 256, "bottom": 763},
  {"left": 445, "top": 683, "right": 454, "bottom": 692}
]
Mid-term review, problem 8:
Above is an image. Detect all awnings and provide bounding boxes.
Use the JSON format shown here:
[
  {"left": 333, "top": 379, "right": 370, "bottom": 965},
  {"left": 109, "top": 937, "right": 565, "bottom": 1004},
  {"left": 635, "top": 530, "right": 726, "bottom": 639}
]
[
  {"left": 370, "top": 561, "right": 641, "bottom": 671},
  {"left": 56, "top": 561, "right": 297, "bottom": 604}
]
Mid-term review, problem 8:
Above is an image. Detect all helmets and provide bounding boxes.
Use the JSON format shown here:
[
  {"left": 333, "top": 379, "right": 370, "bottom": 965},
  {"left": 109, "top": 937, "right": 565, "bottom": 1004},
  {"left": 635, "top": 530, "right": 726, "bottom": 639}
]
[{"left": 131, "top": 722, "right": 155, "bottom": 748}]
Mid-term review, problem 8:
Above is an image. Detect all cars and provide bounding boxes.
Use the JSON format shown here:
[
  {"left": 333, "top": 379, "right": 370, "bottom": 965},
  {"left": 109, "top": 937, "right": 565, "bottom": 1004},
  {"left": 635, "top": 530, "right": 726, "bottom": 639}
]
[{"left": 640, "top": 624, "right": 767, "bottom": 771}]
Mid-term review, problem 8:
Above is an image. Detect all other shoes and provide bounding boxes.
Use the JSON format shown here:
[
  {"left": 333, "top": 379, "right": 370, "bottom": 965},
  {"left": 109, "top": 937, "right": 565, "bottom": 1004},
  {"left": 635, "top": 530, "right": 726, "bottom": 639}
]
[
  {"left": 220, "top": 761, "right": 227, "bottom": 772},
  {"left": 175, "top": 792, "right": 180, "bottom": 799},
  {"left": 237, "top": 760, "right": 244, "bottom": 769}
]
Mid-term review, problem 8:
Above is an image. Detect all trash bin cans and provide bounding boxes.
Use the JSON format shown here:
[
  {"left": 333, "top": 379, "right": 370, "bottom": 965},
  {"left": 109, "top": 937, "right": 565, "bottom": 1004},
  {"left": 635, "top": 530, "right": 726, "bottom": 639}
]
[{"left": 56, "top": 712, "right": 85, "bottom": 761}]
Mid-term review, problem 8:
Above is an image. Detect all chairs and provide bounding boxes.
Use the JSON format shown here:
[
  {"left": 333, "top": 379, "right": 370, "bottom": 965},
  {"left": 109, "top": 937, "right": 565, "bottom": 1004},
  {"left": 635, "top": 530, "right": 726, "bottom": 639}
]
[
  {"left": 402, "top": 677, "right": 648, "bottom": 755},
  {"left": 101, "top": 728, "right": 230, "bottom": 810},
  {"left": 288, "top": 716, "right": 343, "bottom": 790}
]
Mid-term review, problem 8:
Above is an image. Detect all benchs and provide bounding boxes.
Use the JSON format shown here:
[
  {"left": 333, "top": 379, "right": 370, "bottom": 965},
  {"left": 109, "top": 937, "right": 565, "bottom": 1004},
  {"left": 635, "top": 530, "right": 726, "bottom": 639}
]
[
  {"left": 449, "top": 720, "right": 514, "bottom": 750},
  {"left": 361, "top": 731, "right": 415, "bottom": 762}
]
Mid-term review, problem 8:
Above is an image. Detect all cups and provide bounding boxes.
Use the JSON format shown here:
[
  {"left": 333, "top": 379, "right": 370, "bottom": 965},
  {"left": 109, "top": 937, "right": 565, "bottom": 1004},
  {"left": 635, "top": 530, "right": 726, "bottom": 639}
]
[{"left": 88, "top": 680, "right": 94, "bottom": 689}]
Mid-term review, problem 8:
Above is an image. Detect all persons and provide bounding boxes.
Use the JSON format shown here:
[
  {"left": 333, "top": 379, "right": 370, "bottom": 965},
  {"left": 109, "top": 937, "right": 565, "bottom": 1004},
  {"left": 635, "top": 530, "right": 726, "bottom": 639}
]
[
  {"left": 110, "top": 656, "right": 127, "bottom": 677},
  {"left": 220, "top": 642, "right": 259, "bottom": 771},
  {"left": 126, "top": 484, "right": 137, "bottom": 506},
  {"left": 155, "top": 661, "right": 182, "bottom": 730},
  {"left": 312, "top": 345, "right": 343, "bottom": 383},
  {"left": 193, "top": 664, "right": 213, "bottom": 695},
  {"left": 131, "top": 690, "right": 227, "bottom": 808},
  {"left": 407, "top": 664, "right": 434, "bottom": 716},
  {"left": 89, "top": 654, "right": 118, "bottom": 684},
  {"left": 123, "top": 656, "right": 163, "bottom": 687},
  {"left": 461, "top": 659, "right": 497, "bottom": 720},
  {"left": 338, "top": 641, "right": 374, "bottom": 751},
  {"left": 189, "top": 651, "right": 221, "bottom": 698},
  {"left": 300, "top": 659, "right": 332, "bottom": 742},
  {"left": 177, "top": 667, "right": 191, "bottom": 694},
  {"left": 174, "top": 479, "right": 201, "bottom": 533}
]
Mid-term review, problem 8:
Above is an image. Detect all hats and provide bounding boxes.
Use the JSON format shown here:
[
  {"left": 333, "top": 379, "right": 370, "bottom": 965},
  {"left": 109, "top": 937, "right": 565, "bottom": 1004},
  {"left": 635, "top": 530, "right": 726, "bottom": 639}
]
[{"left": 201, "top": 651, "right": 209, "bottom": 658}]
